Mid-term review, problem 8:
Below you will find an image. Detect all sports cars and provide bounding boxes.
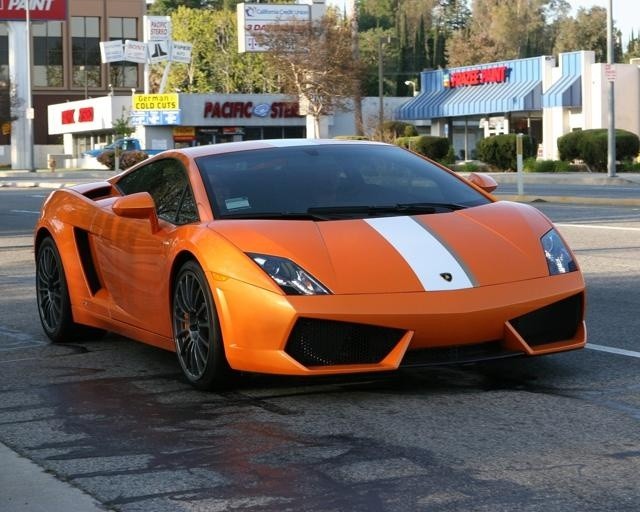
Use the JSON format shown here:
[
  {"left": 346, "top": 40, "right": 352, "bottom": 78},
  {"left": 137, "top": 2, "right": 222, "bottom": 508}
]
[{"left": 33, "top": 136, "right": 590, "bottom": 387}]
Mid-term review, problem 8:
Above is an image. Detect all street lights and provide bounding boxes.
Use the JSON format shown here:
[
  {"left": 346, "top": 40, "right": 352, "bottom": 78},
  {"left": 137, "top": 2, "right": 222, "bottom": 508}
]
[{"left": 404, "top": 79, "right": 417, "bottom": 98}]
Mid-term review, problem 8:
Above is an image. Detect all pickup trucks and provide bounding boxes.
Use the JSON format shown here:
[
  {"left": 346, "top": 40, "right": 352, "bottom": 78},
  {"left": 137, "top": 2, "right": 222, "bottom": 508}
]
[{"left": 80, "top": 138, "right": 167, "bottom": 158}]
